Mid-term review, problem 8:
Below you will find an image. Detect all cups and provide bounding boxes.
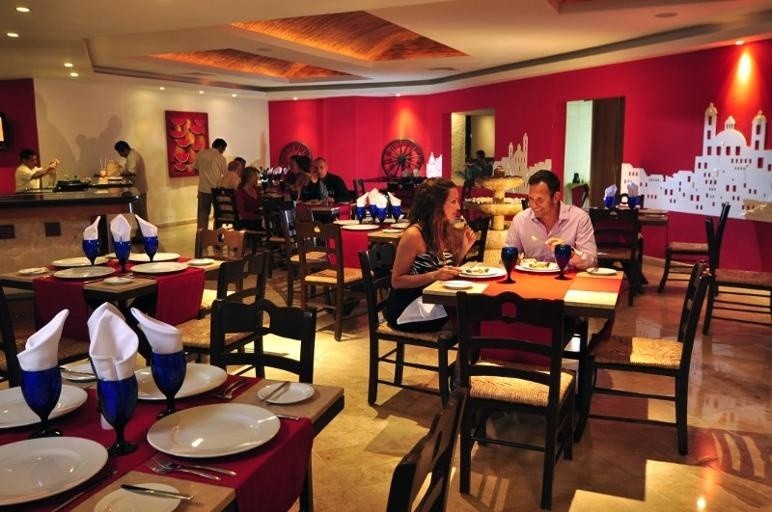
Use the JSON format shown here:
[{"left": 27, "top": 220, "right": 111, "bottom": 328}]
[
  {"left": 148, "top": 348, "right": 189, "bottom": 422},
  {"left": 267, "top": 171, "right": 272, "bottom": 184},
  {"left": 605, "top": 196, "right": 617, "bottom": 217},
  {"left": 111, "top": 240, "right": 134, "bottom": 276},
  {"left": 80, "top": 238, "right": 105, "bottom": 267},
  {"left": 355, "top": 206, "right": 367, "bottom": 224},
  {"left": 500, "top": 245, "right": 520, "bottom": 286},
  {"left": 142, "top": 234, "right": 160, "bottom": 264},
  {"left": 16, "top": 363, "right": 64, "bottom": 439},
  {"left": 553, "top": 243, "right": 573, "bottom": 282},
  {"left": 273, "top": 173, "right": 276, "bottom": 181},
  {"left": 95, "top": 371, "right": 140, "bottom": 458},
  {"left": 262, "top": 181, "right": 267, "bottom": 196},
  {"left": 376, "top": 207, "right": 387, "bottom": 229},
  {"left": 391, "top": 205, "right": 402, "bottom": 224},
  {"left": 277, "top": 173, "right": 281, "bottom": 181},
  {"left": 129, "top": 262, "right": 189, "bottom": 275},
  {"left": 629, "top": 195, "right": 640, "bottom": 215},
  {"left": 322, "top": 191, "right": 329, "bottom": 207},
  {"left": 368, "top": 203, "right": 379, "bottom": 226},
  {"left": 327, "top": 190, "right": 336, "bottom": 207}
]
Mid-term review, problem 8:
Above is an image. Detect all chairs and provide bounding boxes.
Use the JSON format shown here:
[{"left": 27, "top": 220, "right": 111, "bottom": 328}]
[
  {"left": 385, "top": 387, "right": 468, "bottom": 511},
  {"left": 204, "top": 298, "right": 318, "bottom": 512},
  {"left": 281, "top": 207, "right": 339, "bottom": 313},
  {"left": 294, "top": 222, "right": 384, "bottom": 342},
  {"left": 194, "top": 228, "right": 247, "bottom": 365},
  {"left": 467, "top": 215, "right": 493, "bottom": 262},
  {"left": 591, "top": 208, "right": 649, "bottom": 306},
  {"left": 1, "top": 282, "right": 96, "bottom": 387},
  {"left": 659, "top": 202, "right": 731, "bottom": 297},
  {"left": 574, "top": 260, "right": 712, "bottom": 456},
  {"left": 211, "top": 186, "right": 256, "bottom": 254},
  {"left": 455, "top": 290, "right": 577, "bottom": 511},
  {"left": 703, "top": 216, "right": 772, "bottom": 336},
  {"left": 172, "top": 250, "right": 271, "bottom": 379},
  {"left": 358, "top": 241, "right": 472, "bottom": 409},
  {"left": 255, "top": 193, "right": 304, "bottom": 278}
]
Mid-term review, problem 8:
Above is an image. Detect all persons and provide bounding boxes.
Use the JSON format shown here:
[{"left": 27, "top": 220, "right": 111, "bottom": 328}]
[
  {"left": 283, "top": 156, "right": 329, "bottom": 201},
  {"left": 221, "top": 161, "right": 243, "bottom": 218},
  {"left": 196, "top": 138, "right": 228, "bottom": 257},
  {"left": 504, "top": 171, "right": 598, "bottom": 272},
  {"left": 235, "top": 166, "right": 262, "bottom": 229},
  {"left": 292, "top": 156, "right": 312, "bottom": 202},
  {"left": 114, "top": 140, "right": 149, "bottom": 241},
  {"left": 473, "top": 150, "right": 487, "bottom": 165},
  {"left": 289, "top": 155, "right": 301, "bottom": 173},
  {"left": 233, "top": 157, "right": 246, "bottom": 168},
  {"left": 387, "top": 179, "right": 476, "bottom": 333},
  {"left": 301, "top": 157, "right": 350, "bottom": 202},
  {"left": 16, "top": 151, "right": 58, "bottom": 193}
]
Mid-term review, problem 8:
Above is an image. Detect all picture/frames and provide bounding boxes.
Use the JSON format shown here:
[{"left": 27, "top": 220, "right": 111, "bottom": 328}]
[{"left": 165, "top": 109, "right": 211, "bottom": 179}]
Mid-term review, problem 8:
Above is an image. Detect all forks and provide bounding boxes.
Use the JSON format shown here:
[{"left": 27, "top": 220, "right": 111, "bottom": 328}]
[{"left": 144, "top": 453, "right": 240, "bottom": 485}]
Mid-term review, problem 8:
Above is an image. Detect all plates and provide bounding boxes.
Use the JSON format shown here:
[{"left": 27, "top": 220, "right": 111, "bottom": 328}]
[
  {"left": 52, "top": 266, "right": 116, "bottom": 281},
  {"left": 585, "top": 267, "right": 618, "bottom": 276},
  {"left": 0, "top": 434, "right": 112, "bottom": 509},
  {"left": 61, "top": 353, "right": 148, "bottom": 384},
  {"left": 52, "top": 256, "right": 111, "bottom": 268},
  {"left": 442, "top": 278, "right": 476, "bottom": 292},
  {"left": 102, "top": 276, "right": 134, "bottom": 286},
  {"left": 457, "top": 265, "right": 507, "bottom": 280},
  {"left": 127, "top": 251, "right": 180, "bottom": 263},
  {"left": 188, "top": 257, "right": 216, "bottom": 267},
  {"left": 391, "top": 222, "right": 411, "bottom": 229},
  {"left": 17, "top": 267, "right": 48, "bottom": 275},
  {"left": 333, "top": 220, "right": 355, "bottom": 225},
  {"left": 512, "top": 260, "right": 571, "bottom": 274},
  {"left": 145, "top": 399, "right": 284, "bottom": 461},
  {"left": 1, "top": 382, "right": 91, "bottom": 430},
  {"left": 134, "top": 361, "right": 231, "bottom": 402},
  {"left": 90, "top": 479, "right": 185, "bottom": 512},
  {"left": 340, "top": 222, "right": 381, "bottom": 232},
  {"left": 256, "top": 379, "right": 317, "bottom": 407}
]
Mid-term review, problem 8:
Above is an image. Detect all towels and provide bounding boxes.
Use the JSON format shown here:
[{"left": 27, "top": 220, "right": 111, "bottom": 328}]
[
  {"left": 130, "top": 306, "right": 183, "bottom": 355},
  {"left": 274, "top": 168, "right": 276, "bottom": 173},
  {"left": 368, "top": 188, "right": 380, "bottom": 205},
  {"left": 283, "top": 168, "right": 289, "bottom": 174},
  {"left": 82, "top": 216, "right": 102, "bottom": 241},
  {"left": 87, "top": 301, "right": 140, "bottom": 382},
  {"left": 17, "top": 309, "right": 70, "bottom": 372},
  {"left": 356, "top": 191, "right": 369, "bottom": 207},
  {"left": 109, "top": 214, "right": 132, "bottom": 243},
  {"left": 628, "top": 184, "right": 639, "bottom": 197},
  {"left": 278, "top": 166, "right": 282, "bottom": 174},
  {"left": 397, "top": 296, "right": 448, "bottom": 325},
  {"left": 388, "top": 192, "right": 401, "bottom": 206},
  {"left": 374, "top": 193, "right": 387, "bottom": 209},
  {"left": 267, "top": 167, "right": 273, "bottom": 174},
  {"left": 135, "top": 212, "right": 158, "bottom": 239},
  {"left": 605, "top": 184, "right": 618, "bottom": 197}
]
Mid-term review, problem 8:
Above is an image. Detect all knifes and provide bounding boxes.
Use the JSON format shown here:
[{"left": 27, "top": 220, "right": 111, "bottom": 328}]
[{"left": 119, "top": 481, "right": 196, "bottom": 504}]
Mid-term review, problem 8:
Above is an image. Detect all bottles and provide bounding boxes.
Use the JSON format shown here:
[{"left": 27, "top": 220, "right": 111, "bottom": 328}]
[{"left": 320, "top": 186, "right": 328, "bottom": 207}]
